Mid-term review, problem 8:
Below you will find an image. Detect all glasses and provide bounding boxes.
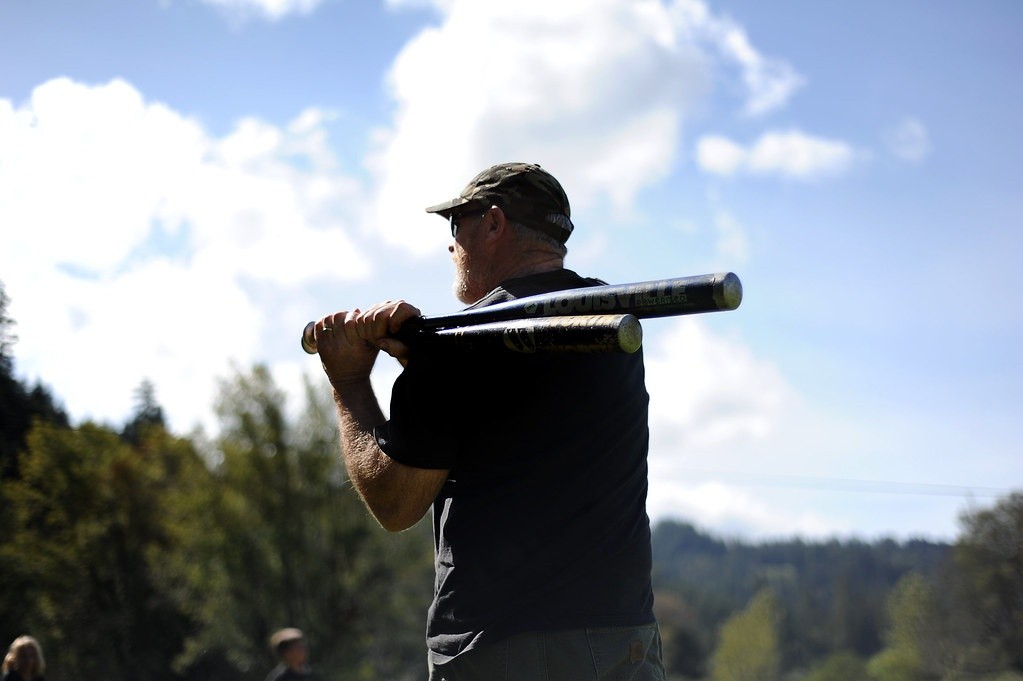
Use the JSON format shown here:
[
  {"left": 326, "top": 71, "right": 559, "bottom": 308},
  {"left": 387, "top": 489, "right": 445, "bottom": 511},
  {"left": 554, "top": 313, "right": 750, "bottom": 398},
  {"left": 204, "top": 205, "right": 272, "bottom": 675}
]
[{"left": 450, "top": 211, "right": 481, "bottom": 238}]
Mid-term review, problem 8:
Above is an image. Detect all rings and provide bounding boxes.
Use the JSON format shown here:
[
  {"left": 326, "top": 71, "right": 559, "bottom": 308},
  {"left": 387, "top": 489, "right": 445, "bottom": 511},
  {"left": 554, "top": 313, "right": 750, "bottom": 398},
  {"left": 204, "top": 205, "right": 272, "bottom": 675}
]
[{"left": 323, "top": 328, "right": 332, "bottom": 331}]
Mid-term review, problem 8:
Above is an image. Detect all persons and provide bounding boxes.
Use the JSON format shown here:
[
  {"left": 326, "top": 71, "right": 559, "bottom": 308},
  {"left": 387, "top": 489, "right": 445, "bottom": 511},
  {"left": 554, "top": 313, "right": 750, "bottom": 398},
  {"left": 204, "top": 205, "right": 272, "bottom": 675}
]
[
  {"left": 0, "top": 636, "right": 45, "bottom": 681},
  {"left": 313, "top": 163, "right": 667, "bottom": 681},
  {"left": 267, "top": 629, "right": 313, "bottom": 681}
]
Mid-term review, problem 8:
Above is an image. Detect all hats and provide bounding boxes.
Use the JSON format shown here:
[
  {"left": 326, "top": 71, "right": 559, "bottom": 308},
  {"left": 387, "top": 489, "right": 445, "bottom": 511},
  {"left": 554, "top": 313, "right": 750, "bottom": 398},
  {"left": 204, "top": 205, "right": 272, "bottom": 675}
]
[{"left": 428, "top": 162, "right": 574, "bottom": 245}]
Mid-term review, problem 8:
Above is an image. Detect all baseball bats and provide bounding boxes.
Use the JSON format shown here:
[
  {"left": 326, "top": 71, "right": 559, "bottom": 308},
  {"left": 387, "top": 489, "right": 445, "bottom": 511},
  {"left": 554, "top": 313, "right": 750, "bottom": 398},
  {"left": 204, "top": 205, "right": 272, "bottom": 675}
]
[
  {"left": 303, "top": 269, "right": 743, "bottom": 347},
  {"left": 301, "top": 314, "right": 643, "bottom": 356}
]
